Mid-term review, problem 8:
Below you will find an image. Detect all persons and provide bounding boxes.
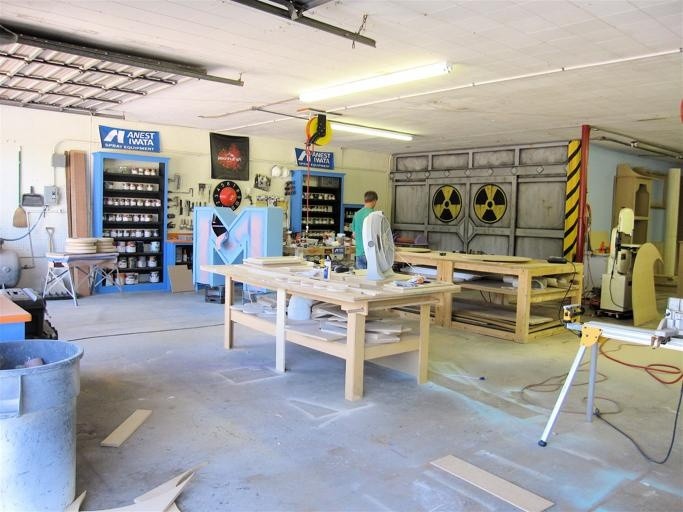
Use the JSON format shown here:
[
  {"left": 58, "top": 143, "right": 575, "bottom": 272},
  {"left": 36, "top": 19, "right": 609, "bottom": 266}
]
[{"left": 352, "top": 191, "right": 378, "bottom": 270}]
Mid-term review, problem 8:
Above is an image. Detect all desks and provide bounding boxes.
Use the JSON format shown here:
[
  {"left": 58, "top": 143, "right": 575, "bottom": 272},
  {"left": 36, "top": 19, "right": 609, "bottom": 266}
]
[
  {"left": 42, "top": 253, "right": 125, "bottom": 307},
  {"left": 201, "top": 259, "right": 461, "bottom": 401},
  {"left": 393, "top": 246, "right": 584, "bottom": 344}
]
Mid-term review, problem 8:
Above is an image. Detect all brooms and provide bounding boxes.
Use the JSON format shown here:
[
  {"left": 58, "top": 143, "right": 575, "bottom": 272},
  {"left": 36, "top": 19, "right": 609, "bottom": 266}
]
[{"left": 12, "top": 146, "right": 27, "bottom": 227}]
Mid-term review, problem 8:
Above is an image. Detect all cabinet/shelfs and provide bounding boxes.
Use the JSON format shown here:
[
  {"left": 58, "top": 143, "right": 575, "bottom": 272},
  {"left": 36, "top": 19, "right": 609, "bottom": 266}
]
[
  {"left": 91, "top": 152, "right": 171, "bottom": 295},
  {"left": 289, "top": 169, "right": 364, "bottom": 238},
  {"left": 631, "top": 178, "right": 650, "bottom": 245}
]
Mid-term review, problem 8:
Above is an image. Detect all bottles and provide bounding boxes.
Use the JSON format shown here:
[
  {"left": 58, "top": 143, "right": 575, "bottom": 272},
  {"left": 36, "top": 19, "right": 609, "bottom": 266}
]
[
  {"left": 183, "top": 249, "right": 187, "bottom": 264},
  {"left": 323, "top": 256, "right": 332, "bottom": 281}
]
